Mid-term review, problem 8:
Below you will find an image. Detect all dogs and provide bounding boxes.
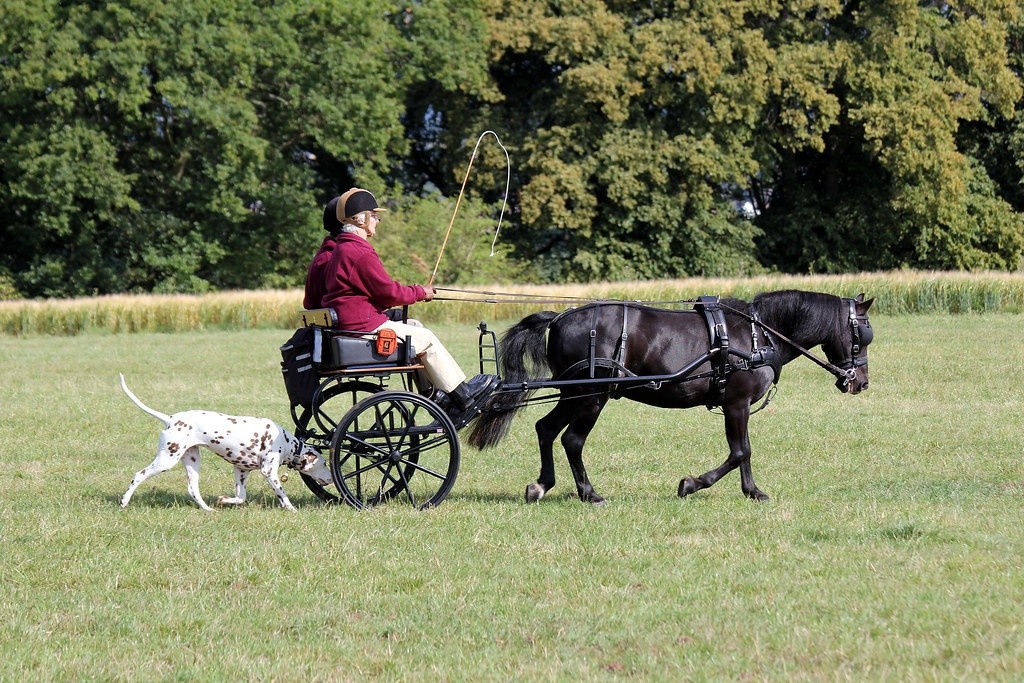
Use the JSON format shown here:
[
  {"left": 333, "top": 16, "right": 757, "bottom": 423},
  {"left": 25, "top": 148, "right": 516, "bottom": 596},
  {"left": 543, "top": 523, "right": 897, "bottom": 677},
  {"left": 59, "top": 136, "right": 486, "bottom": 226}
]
[{"left": 118, "top": 372, "right": 334, "bottom": 512}]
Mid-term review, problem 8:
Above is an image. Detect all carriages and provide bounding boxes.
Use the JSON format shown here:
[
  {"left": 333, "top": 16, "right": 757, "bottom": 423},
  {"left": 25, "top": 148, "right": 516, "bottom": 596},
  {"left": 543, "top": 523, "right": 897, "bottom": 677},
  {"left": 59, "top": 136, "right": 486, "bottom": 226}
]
[{"left": 277, "top": 282, "right": 877, "bottom": 518}]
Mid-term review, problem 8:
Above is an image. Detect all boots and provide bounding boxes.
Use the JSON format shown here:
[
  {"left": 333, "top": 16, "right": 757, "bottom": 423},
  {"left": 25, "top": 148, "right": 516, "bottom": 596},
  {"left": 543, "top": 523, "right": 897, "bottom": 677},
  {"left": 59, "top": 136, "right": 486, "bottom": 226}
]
[
  {"left": 453, "top": 374, "right": 492, "bottom": 410},
  {"left": 428, "top": 389, "right": 453, "bottom": 421}
]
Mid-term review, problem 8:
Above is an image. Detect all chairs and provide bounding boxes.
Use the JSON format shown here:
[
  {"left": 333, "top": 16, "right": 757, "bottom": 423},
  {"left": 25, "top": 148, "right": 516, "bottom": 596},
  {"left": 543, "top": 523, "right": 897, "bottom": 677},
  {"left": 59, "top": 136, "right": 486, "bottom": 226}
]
[{"left": 299, "top": 308, "right": 417, "bottom": 369}]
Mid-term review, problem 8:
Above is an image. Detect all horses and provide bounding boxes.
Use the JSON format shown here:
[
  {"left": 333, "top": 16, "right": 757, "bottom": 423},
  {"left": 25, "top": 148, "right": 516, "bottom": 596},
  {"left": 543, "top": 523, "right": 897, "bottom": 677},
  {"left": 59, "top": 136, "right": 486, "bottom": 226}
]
[{"left": 459, "top": 289, "right": 877, "bottom": 505}]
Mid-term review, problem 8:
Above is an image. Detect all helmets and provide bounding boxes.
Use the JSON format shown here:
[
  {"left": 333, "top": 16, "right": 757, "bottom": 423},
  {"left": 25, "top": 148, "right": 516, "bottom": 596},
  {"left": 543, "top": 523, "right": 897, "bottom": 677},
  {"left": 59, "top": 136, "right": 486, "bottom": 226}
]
[
  {"left": 322, "top": 196, "right": 344, "bottom": 231},
  {"left": 336, "top": 187, "right": 388, "bottom": 219}
]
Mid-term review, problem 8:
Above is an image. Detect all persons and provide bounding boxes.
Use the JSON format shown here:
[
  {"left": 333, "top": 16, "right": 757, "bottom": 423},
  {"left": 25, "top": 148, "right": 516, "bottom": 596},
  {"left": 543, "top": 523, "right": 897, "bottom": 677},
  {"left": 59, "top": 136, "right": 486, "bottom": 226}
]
[
  {"left": 321, "top": 187, "right": 494, "bottom": 416},
  {"left": 303, "top": 195, "right": 453, "bottom": 414}
]
